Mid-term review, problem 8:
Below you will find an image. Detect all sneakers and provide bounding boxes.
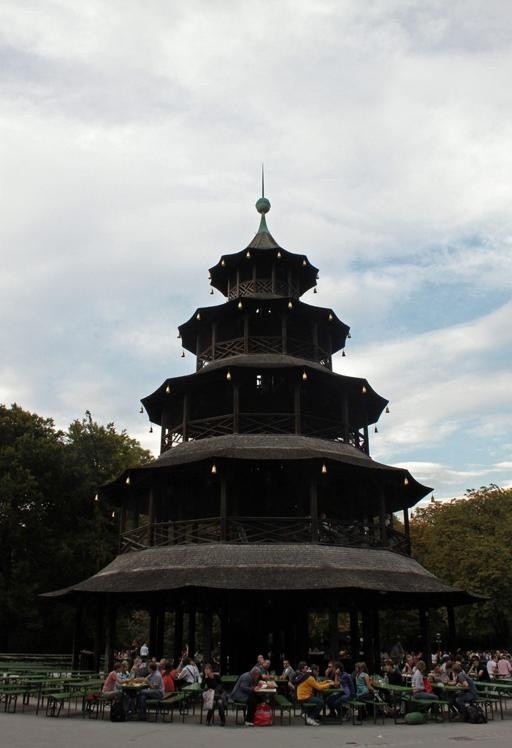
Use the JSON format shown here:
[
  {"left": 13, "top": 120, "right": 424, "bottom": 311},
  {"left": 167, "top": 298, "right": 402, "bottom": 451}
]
[
  {"left": 245, "top": 721, "right": 254, "bottom": 726},
  {"left": 301, "top": 712, "right": 319, "bottom": 726}
]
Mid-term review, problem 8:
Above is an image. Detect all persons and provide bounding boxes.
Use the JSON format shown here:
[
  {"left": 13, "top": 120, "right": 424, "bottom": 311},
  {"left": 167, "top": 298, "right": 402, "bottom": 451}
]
[{"left": 102, "top": 637, "right": 512, "bottom": 726}]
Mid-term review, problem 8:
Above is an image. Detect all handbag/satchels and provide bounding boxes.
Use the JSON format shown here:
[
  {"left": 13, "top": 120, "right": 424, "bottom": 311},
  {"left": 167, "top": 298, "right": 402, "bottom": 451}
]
[
  {"left": 465, "top": 704, "right": 488, "bottom": 724},
  {"left": 202, "top": 688, "right": 215, "bottom": 711}
]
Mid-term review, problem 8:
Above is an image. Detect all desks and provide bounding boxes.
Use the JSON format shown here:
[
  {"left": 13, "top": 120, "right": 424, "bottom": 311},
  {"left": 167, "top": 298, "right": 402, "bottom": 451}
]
[{"left": 0, "top": 670, "right": 511, "bottom": 726}]
